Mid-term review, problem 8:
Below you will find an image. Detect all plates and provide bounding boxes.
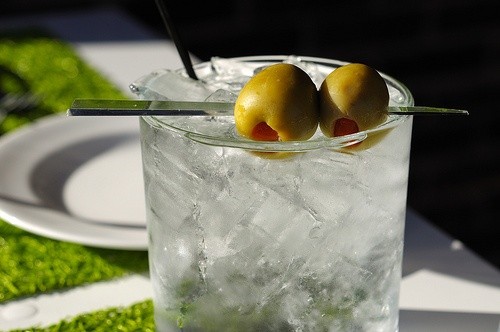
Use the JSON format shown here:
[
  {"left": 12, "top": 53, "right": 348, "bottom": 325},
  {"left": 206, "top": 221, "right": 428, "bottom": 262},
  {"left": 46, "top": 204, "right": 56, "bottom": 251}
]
[{"left": 1, "top": 109, "right": 147, "bottom": 252}]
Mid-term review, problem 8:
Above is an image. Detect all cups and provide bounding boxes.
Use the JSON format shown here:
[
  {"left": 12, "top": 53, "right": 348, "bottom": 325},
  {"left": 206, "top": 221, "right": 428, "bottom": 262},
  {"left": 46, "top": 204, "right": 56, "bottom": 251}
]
[{"left": 138, "top": 53, "right": 415, "bottom": 332}]
